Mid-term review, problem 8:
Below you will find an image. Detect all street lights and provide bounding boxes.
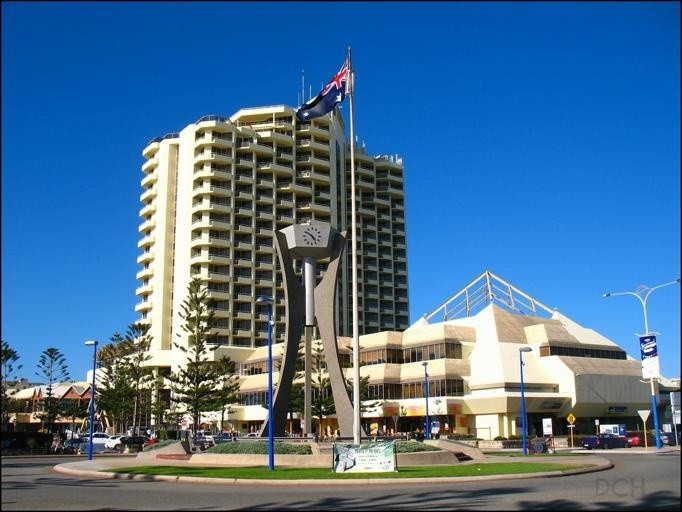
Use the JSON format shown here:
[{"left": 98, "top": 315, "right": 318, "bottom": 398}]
[
  {"left": 603, "top": 279, "right": 681, "bottom": 448},
  {"left": 422, "top": 361, "right": 430, "bottom": 439},
  {"left": 519, "top": 347, "right": 532, "bottom": 455},
  {"left": 255, "top": 296, "right": 275, "bottom": 470},
  {"left": 84, "top": 341, "right": 98, "bottom": 459}
]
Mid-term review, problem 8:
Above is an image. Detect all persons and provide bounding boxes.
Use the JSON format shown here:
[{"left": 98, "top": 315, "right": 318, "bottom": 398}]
[
  {"left": 373, "top": 427, "right": 379, "bottom": 442},
  {"left": 416, "top": 427, "right": 424, "bottom": 443}
]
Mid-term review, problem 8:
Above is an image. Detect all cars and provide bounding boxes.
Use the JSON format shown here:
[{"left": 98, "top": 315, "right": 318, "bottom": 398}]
[
  {"left": 189, "top": 431, "right": 238, "bottom": 442},
  {"left": 584, "top": 431, "right": 681, "bottom": 450},
  {"left": 82, "top": 433, "right": 159, "bottom": 452}
]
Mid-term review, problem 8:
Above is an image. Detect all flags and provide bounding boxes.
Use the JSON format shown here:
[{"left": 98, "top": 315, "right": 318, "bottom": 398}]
[
  {"left": 639, "top": 336, "right": 661, "bottom": 380},
  {"left": 293, "top": 58, "right": 350, "bottom": 121}
]
